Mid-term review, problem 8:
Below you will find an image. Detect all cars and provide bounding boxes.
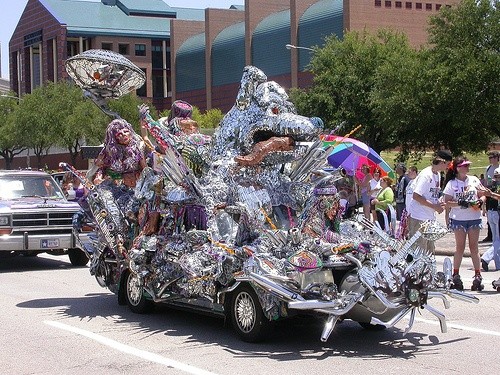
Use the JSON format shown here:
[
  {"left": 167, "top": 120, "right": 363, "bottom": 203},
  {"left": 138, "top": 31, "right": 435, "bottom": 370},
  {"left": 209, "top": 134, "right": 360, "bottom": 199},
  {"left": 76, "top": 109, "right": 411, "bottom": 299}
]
[{"left": 0, "top": 169, "right": 92, "bottom": 268}]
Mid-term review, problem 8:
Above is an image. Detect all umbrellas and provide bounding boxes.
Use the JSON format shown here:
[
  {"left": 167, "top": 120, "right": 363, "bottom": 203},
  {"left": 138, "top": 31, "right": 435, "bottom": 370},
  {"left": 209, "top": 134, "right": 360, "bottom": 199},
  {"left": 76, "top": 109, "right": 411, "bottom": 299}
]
[{"left": 319, "top": 134, "right": 394, "bottom": 182}]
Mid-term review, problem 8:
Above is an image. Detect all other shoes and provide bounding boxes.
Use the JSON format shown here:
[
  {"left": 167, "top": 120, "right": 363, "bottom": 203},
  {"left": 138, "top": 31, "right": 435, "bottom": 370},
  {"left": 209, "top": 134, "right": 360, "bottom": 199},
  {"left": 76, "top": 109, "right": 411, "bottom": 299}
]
[
  {"left": 481, "top": 259, "right": 488, "bottom": 271},
  {"left": 492, "top": 277, "right": 500, "bottom": 292}
]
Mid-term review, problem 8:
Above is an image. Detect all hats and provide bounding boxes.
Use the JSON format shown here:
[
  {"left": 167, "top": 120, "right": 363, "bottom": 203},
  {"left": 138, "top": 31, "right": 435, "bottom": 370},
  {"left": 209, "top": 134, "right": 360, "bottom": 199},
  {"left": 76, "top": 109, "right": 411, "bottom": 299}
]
[
  {"left": 494, "top": 168, "right": 500, "bottom": 178},
  {"left": 430, "top": 150, "right": 453, "bottom": 162},
  {"left": 456, "top": 160, "right": 473, "bottom": 168}
]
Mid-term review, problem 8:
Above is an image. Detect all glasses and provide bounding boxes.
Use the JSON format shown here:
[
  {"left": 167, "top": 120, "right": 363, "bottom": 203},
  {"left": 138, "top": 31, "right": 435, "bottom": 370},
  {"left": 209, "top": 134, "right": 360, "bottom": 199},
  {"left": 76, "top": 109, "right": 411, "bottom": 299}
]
[
  {"left": 463, "top": 164, "right": 470, "bottom": 167},
  {"left": 489, "top": 157, "right": 494, "bottom": 159}
]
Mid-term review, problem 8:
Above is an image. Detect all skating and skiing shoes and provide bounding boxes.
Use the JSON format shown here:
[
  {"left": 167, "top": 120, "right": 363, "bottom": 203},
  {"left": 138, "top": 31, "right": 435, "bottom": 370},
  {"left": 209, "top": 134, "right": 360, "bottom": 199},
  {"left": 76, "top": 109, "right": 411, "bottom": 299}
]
[
  {"left": 450, "top": 274, "right": 463, "bottom": 290},
  {"left": 471, "top": 275, "right": 484, "bottom": 292}
]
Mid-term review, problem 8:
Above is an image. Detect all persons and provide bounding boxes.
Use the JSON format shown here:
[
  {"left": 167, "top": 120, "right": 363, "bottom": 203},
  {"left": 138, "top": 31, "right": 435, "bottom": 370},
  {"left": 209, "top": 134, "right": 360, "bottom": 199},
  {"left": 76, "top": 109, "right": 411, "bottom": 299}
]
[
  {"left": 299, "top": 182, "right": 371, "bottom": 256},
  {"left": 86, "top": 119, "right": 151, "bottom": 234},
  {"left": 0, "top": 139, "right": 500, "bottom": 294}
]
